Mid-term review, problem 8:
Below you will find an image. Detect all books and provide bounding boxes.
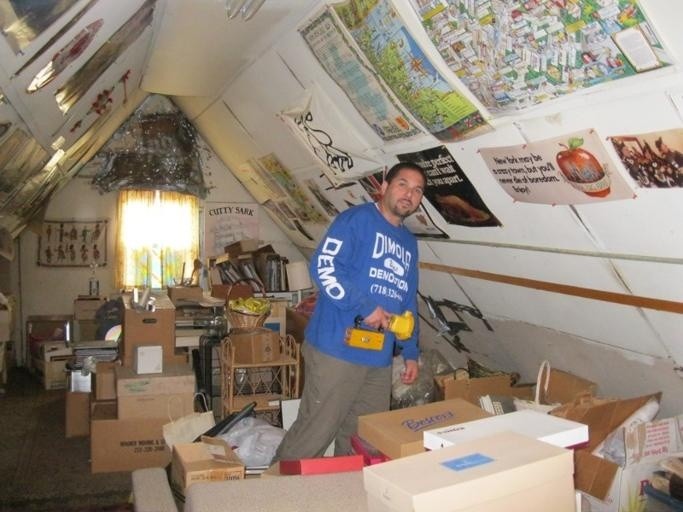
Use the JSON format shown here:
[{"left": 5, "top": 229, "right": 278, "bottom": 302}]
[
  {"left": 218, "top": 255, "right": 287, "bottom": 293},
  {"left": 479, "top": 394, "right": 515, "bottom": 415}
]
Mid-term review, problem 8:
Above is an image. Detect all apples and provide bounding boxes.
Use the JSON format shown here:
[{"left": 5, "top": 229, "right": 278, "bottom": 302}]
[{"left": 556, "top": 137, "right": 612, "bottom": 197}]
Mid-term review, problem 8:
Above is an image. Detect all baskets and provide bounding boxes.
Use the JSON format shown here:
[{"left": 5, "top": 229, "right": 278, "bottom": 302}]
[{"left": 222, "top": 276, "right": 272, "bottom": 332}]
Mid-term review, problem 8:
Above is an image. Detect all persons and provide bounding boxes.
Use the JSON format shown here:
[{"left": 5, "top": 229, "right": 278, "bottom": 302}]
[
  {"left": 268, "top": 161, "right": 427, "bottom": 468},
  {"left": 619, "top": 139, "right": 683, "bottom": 188}
]
